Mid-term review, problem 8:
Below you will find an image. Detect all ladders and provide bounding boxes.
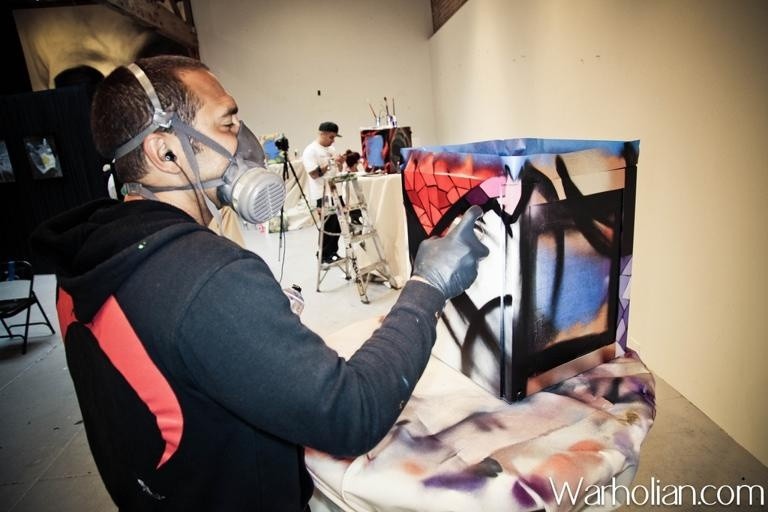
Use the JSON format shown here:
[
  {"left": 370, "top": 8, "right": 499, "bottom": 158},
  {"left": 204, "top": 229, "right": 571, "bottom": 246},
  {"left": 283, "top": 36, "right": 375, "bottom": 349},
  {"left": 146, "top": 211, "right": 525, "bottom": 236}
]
[{"left": 317, "top": 174, "right": 403, "bottom": 305}]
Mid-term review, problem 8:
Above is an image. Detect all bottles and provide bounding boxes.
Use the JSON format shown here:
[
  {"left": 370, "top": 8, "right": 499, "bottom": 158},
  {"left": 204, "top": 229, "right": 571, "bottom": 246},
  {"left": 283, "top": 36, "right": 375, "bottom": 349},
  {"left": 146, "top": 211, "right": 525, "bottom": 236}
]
[
  {"left": 445, "top": 214, "right": 485, "bottom": 249},
  {"left": 279, "top": 283, "right": 306, "bottom": 317},
  {"left": 328, "top": 155, "right": 337, "bottom": 177}
]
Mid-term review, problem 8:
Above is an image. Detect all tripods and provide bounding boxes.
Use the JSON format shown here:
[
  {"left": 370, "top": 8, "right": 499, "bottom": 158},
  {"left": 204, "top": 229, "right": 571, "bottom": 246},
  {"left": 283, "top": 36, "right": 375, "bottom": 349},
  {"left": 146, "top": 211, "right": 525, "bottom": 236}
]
[{"left": 276, "top": 151, "right": 322, "bottom": 261}]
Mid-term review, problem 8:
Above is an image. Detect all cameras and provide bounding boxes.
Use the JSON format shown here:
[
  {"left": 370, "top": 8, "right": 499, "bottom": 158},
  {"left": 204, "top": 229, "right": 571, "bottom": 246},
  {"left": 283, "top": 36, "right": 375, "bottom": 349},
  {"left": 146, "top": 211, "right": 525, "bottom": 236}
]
[{"left": 274, "top": 136, "right": 289, "bottom": 150}]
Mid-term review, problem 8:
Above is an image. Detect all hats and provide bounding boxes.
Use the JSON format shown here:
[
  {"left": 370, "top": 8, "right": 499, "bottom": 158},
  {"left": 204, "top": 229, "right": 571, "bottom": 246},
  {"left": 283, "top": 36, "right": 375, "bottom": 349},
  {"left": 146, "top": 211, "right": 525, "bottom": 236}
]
[{"left": 319, "top": 122, "right": 342, "bottom": 138}]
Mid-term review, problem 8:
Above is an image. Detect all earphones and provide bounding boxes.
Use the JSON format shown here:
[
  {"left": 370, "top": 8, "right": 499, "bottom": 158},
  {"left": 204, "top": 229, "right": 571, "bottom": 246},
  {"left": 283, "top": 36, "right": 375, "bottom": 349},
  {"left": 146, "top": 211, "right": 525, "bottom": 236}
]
[{"left": 165, "top": 151, "right": 175, "bottom": 163}]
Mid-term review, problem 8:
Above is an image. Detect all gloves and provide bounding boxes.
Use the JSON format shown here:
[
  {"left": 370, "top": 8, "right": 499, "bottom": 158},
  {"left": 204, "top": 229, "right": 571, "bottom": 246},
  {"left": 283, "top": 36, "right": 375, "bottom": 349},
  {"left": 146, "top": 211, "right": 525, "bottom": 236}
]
[{"left": 410, "top": 205, "right": 489, "bottom": 302}]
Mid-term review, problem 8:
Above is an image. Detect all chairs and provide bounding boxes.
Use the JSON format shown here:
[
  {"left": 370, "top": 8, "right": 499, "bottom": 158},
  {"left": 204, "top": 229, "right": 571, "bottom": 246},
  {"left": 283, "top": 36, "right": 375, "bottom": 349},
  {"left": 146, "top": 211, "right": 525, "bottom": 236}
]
[{"left": 0, "top": 261, "right": 54, "bottom": 354}]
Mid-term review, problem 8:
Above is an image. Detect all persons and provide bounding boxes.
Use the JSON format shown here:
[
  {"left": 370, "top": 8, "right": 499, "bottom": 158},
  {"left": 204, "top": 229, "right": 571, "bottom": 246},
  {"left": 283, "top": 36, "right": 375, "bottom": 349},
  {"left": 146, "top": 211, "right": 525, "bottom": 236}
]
[
  {"left": 52, "top": 52, "right": 484, "bottom": 512},
  {"left": 302, "top": 121, "right": 349, "bottom": 262}
]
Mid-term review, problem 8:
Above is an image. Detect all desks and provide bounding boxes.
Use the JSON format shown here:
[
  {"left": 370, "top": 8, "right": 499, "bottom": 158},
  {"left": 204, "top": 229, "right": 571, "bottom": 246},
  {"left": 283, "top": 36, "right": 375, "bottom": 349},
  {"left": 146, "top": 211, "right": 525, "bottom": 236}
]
[
  {"left": 269, "top": 161, "right": 402, "bottom": 290},
  {"left": 304, "top": 299, "right": 766, "bottom": 508}
]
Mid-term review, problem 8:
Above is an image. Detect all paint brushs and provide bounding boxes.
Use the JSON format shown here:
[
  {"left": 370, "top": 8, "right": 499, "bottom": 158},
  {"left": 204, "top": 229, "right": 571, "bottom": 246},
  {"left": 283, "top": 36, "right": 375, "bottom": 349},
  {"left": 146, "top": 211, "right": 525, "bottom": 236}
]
[
  {"left": 367, "top": 99, "right": 376, "bottom": 118},
  {"left": 392, "top": 98, "right": 395, "bottom": 115},
  {"left": 384, "top": 97, "right": 388, "bottom": 115}
]
